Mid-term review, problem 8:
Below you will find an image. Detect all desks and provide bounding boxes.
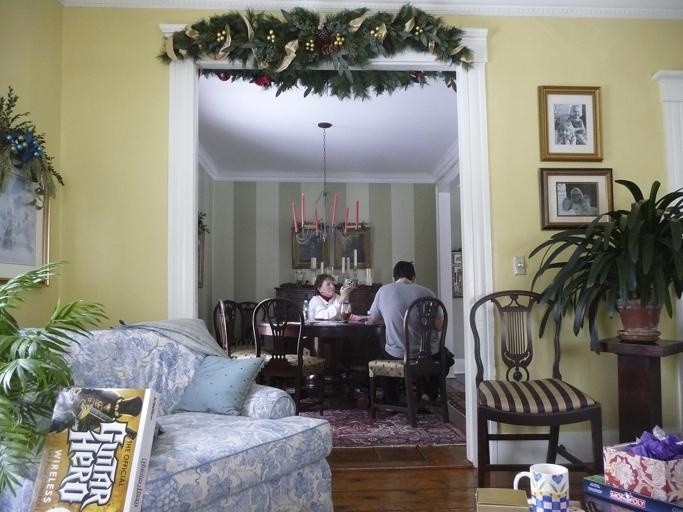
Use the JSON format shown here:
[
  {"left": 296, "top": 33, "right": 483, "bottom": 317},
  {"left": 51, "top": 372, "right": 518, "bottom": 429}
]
[{"left": 590, "top": 335, "right": 680, "bottom": 451}]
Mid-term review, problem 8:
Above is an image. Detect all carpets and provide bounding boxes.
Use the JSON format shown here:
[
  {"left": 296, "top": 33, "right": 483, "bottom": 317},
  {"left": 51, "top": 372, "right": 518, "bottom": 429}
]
[
  {"left": 441, "top": 370, "right": 465, "bottom": 417},
  {"left": 288, "top": 379, "right": 469, "bottom": 450}
]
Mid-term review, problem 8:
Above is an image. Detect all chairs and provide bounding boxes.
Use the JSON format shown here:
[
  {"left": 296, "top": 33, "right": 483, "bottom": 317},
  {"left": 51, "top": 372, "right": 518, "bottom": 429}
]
[
  {"left": 0, "top": 314, "right": 335, "bottom": 510},
  {"left": 210, "top": 295, "right": 452, "bottom": 429},
  {"left": 469, "top": 288, "right": 604, "bottom": 487}
]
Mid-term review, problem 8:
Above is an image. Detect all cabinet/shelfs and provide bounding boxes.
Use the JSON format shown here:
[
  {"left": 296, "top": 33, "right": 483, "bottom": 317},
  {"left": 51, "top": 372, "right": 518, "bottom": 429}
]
[{"left": 273, "top": 280, "right": 381, "bottom": 320}]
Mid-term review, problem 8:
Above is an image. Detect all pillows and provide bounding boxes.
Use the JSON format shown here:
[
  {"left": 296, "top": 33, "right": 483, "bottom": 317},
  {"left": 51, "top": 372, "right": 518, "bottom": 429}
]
[{"left": 174, "top": 350, "right": 267, "bottom": 418}]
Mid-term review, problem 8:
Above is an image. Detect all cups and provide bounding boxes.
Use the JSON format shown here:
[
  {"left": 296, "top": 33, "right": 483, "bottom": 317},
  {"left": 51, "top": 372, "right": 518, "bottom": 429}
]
[
  {"left": 513, "top": 463, "right": 570, "bottom": 512},
  {"left": 364, "top": 268, "right": 373, "bottom": 287}
]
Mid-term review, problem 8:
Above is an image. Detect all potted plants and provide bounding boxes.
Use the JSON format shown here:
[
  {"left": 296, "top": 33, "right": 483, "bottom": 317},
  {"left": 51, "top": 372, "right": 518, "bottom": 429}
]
[{"left": 527, "top": 172, "right": 683, "bottom": 356}]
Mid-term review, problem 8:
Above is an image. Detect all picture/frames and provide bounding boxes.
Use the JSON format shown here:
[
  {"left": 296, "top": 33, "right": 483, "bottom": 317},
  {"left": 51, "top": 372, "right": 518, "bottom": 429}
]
[
  {"left": 289, "top": 220, "right": 329, "bottom": 270},
  {"left": 0, "top": 139, "right": 52, "bottom": 292},
  {"left": 335, "top": 223, "right": 371, "bottom": 268},
  {"left": 537, "top": 81, "right": 603, "bottom": 164},
  {"left": 536, "top": 165, "right": 615, "bottom": 228}
]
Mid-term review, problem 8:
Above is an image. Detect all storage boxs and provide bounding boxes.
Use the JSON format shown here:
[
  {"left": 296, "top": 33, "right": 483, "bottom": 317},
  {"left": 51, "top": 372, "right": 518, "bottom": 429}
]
[
  {"left": 602, "top": 438, "right": 682, "bottom": 503},
  {"left": 30, "top": 380, "right": 161, "bottom": 512}
]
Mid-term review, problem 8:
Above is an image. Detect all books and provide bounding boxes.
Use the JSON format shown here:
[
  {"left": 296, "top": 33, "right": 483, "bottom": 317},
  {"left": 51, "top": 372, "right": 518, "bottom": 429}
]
[
  {"left": 475, "top": 487, "right": 530, "bottom": 512},
  {"left": 583, "top": 474, "right": 683, "bottom": 512}
]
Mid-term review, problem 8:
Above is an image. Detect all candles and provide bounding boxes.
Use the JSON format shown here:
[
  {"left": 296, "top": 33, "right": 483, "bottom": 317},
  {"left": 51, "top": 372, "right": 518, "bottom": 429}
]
[
  {"left": 292, "top": 248, "right": 359, "bottom": 282},
  {"left": 290, "top": 192, "right": 362, "bottom": 235}
]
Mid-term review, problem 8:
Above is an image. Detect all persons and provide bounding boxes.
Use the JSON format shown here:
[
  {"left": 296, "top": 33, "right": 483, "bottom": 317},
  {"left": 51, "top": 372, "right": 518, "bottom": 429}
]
[
  {"left": 367, "top": 261, "right": 455, "bottom": 414},
  {"left": 568, "top": 105, "right": 587, "bottom": 145},
  {"left": 308, "top": 274, "right": 353, "bottom": 321}
]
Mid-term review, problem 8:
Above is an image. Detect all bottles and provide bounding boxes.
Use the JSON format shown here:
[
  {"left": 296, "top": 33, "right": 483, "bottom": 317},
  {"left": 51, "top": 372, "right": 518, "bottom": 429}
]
[
  {"left": 302, "top": 300, "right": 308, "bottom": 323},
  {"left": 366, "top": 311, "right": 370, "bottom": 320}
]
